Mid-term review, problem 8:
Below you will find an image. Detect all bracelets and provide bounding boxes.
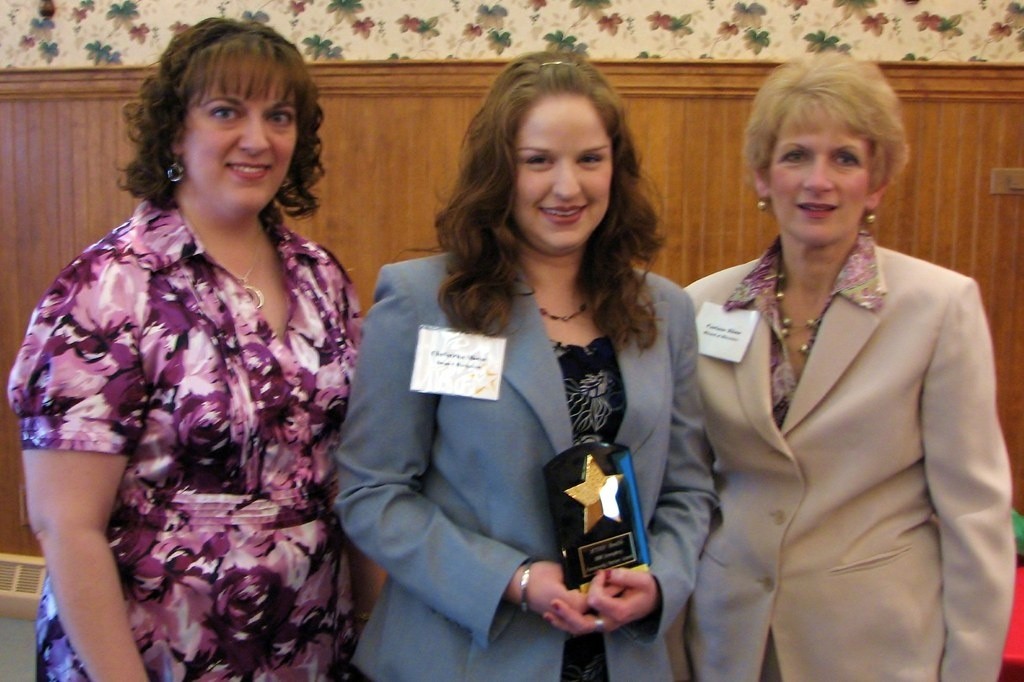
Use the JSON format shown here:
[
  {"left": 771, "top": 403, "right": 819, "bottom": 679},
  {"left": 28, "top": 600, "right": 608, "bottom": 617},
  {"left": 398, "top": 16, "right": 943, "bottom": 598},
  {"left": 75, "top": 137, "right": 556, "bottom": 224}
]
[{"left": 521, "top": 562, "right": 534, "bottom": 613}]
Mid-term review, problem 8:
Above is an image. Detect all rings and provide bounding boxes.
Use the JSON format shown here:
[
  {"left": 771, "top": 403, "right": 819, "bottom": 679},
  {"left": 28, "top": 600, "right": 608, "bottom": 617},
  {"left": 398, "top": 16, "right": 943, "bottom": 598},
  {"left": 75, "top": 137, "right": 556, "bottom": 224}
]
[{"left": 596, "top": 618, "right": 605, "bottom": 630}]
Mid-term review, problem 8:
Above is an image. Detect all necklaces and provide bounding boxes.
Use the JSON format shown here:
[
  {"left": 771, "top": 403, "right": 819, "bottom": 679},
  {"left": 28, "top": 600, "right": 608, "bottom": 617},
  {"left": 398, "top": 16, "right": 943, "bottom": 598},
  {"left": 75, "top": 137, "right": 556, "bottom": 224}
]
[
  {"left": 538, "top": 301, "right": 587, "bottom": 321},
  {"left": 774, "top": 281, "right": 819, "bottom": 355},
  {"left": 208, "top": 228, "right": 266, "bottom": 314}
]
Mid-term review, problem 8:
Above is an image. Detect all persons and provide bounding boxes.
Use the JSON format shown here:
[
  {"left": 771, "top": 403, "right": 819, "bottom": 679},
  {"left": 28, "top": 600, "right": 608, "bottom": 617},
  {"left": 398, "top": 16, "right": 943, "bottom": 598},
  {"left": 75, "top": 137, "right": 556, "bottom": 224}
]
[
  {"left": 5, "top": 18, "right": 385, "bottom": 682},
  {"left": 681, "top": 47, "right": 1016, "bottom": 682},
  {"left": 333, "top": 53, "right": 718, "bottom": 682}
]
